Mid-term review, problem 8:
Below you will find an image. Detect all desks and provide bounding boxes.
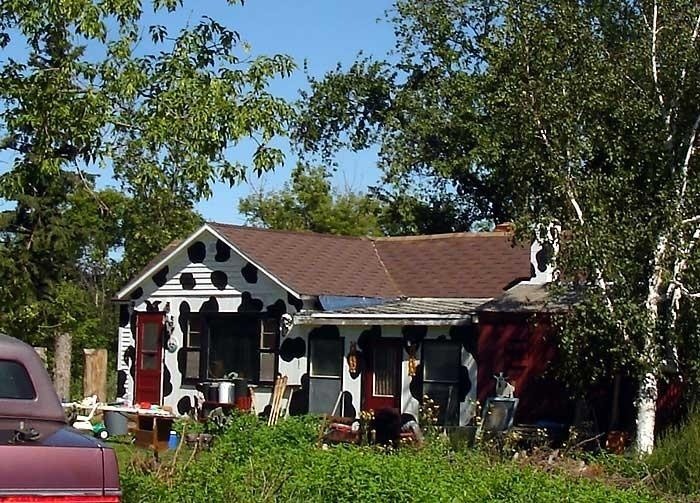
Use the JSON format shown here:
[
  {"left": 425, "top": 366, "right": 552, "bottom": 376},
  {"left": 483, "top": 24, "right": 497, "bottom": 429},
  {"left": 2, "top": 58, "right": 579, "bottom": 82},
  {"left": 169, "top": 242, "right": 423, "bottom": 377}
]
[{"left": 61, "top": 400, "right": 179, "bottom": 460}]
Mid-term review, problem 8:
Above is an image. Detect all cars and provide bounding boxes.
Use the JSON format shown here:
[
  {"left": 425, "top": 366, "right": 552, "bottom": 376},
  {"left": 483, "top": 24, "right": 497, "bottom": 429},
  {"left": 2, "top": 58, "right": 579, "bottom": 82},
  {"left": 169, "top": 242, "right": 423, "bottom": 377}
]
[{"left": 0, "top": 332, "right": 122, "bottom": 503}]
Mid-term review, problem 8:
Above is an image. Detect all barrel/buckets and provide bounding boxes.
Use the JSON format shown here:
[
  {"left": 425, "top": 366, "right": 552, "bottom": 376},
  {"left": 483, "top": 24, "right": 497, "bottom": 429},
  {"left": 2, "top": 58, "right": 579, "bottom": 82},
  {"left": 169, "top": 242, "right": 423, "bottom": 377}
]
[{"left": 105, "top": 412, "right": 128, "bottom": 436}]
